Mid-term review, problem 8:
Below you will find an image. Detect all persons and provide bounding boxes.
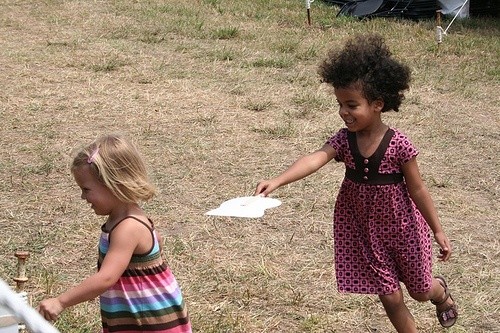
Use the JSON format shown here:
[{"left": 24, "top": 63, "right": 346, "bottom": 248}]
[
  {"left": 255, "top": 33, "right": 459, "bottom": 333},
  {"left": 37, "top": 135, "right": 194, "bottom": 333}
]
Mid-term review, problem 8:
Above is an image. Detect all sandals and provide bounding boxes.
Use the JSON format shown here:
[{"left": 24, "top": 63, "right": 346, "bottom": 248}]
[{"left": 430, "top": 275, "right": 457, "bottom": 327}]
